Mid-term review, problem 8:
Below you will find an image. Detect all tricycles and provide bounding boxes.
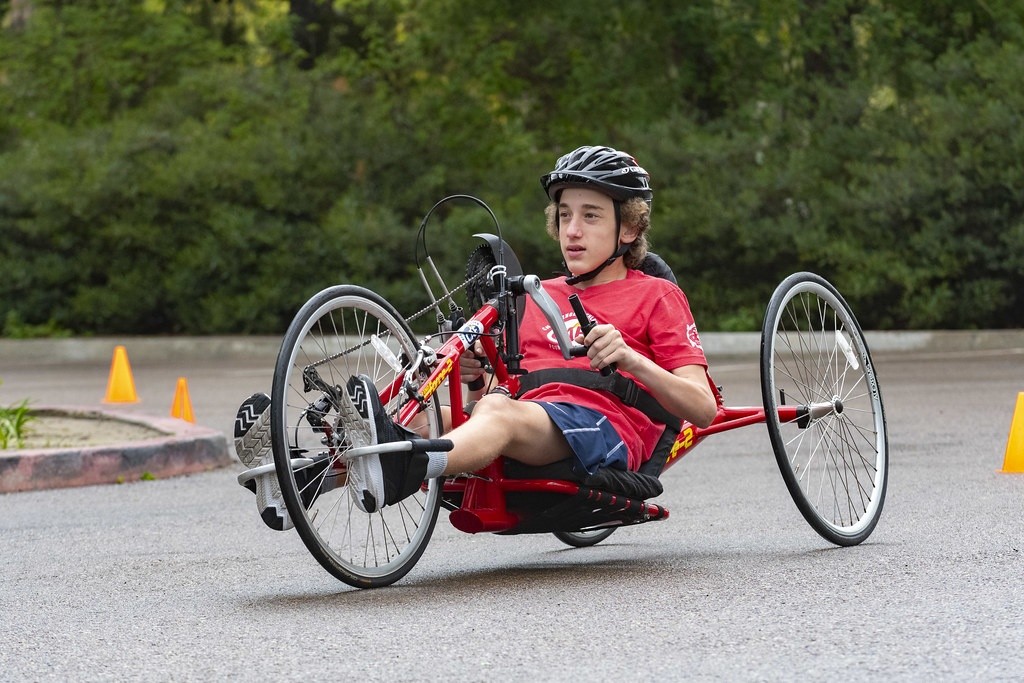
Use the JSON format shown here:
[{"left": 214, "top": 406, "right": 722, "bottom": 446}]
[{"left": 237, "top": 197, "right": 889, "bottom": 591}]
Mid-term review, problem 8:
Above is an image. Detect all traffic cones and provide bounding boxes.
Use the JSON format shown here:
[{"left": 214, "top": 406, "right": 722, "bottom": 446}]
[
  {"left": 991, "top": 392, "right": 1024, "bottom": 474},
  {"left": 100, "top": 346, "right": 140, "bottom": 406},
  {"left": 171, "top": 377, "right": 199, "bottom": 425}
]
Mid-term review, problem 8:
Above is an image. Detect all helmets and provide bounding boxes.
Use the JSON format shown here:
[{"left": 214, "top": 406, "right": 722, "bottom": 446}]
[{"left": 541, "top": 146, "right": 652, "bottom": 228}]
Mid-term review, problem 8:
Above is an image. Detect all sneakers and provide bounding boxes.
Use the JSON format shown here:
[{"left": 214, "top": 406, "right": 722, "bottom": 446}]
[
  {"left": 341, "top": 373, "right": 428, "bottom": 513},
  {"left": 233, "top": 392, "right": 323, "bottom": 531}
]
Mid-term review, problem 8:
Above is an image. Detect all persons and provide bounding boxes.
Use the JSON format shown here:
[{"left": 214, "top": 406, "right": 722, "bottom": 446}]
[{"left": 234, "top": 145, "right": 718, "bottom": 531}]
